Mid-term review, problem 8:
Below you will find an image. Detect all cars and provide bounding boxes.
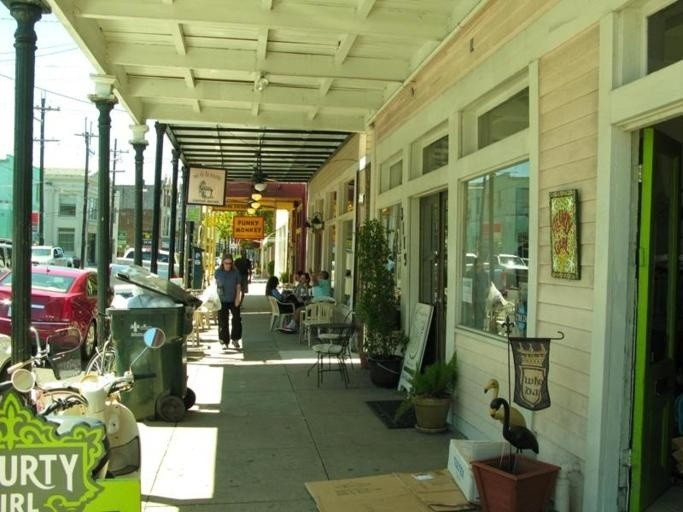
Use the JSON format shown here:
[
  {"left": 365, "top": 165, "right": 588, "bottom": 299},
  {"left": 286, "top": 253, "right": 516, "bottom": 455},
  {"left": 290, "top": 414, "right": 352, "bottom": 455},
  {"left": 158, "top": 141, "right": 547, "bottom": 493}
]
[
  {"left": 465, "top": 252, "right": 529, "bottom": 278},
  {"left": 0, "top": 264, "right": 114, "bottom": 357},
  {"left": 0, "top": 334, "right": 28, "bottom": 396},
  {"left": 0, "top": 239, "right": 12, "bottom": 268}
]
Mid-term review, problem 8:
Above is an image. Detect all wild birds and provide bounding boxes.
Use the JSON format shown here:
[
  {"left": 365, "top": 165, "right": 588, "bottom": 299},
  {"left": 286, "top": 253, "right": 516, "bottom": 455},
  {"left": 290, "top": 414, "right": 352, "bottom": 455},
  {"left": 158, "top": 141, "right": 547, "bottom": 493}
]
[
  {"left": 489, "top": 397, "right": 539, "bottom": 474},
  {"left": 483, "top": 378, "right": 527, "bottom": 469}
]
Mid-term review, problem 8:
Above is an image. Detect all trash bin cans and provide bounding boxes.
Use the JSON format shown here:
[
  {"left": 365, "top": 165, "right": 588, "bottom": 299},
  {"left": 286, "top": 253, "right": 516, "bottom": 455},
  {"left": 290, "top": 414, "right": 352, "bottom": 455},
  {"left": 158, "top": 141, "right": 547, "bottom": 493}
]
[{"left": 105, "top": 264, "right": 202, "bottom": 423}]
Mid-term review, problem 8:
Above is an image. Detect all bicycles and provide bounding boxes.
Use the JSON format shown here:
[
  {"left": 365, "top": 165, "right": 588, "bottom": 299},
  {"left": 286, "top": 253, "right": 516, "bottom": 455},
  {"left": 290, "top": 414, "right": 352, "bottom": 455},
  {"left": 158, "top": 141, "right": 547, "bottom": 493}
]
[
  {"left": 85, "top": 333, "right": 120, "bottom": 380},
  {"left": 4, "top": 317, "right": 84, "bottom": 388}
]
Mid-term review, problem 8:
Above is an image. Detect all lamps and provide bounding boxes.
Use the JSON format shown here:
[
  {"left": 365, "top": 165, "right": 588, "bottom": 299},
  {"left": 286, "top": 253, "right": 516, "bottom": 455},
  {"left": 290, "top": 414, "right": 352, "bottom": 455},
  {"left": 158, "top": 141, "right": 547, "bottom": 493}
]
[
  {"left": 247, "top": 138, "right": 267, "bottom": 215},
  {"left": 304, "top": 222, "right": 314, "bottom": 232},
  {"left": 255, "top": 72, "right": 269, "bottom": 92},
  {"left": 311, "top": 212, "right": 324, "bottom": 230}
]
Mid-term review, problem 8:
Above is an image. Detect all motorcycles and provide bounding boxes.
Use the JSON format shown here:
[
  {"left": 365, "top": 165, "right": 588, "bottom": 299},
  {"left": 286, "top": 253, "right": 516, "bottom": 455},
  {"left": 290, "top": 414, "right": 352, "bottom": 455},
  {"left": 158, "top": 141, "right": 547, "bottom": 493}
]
[{"left": 12, "top": 325, "right": 165, "bottom": 512}]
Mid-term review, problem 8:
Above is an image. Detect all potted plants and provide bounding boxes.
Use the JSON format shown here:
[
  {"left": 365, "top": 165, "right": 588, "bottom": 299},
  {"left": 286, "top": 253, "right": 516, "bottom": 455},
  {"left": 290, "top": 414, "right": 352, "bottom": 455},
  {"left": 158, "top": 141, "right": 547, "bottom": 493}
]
[
  {"left": 391, "top": 351, "right": 458, "bottom": 434},
  {"left": 342, "top": 217, "right": 409, "bottom": 390}
]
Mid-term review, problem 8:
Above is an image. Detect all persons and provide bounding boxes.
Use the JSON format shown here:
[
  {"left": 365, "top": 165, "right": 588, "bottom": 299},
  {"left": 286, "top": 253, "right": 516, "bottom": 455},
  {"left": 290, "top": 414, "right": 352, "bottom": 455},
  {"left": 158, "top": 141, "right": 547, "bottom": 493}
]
[
  {"left": 471, "top": 254, "right": 492, "bottom": 331},
  {"left": 233, "top": 249, "right": 252, "bottom": 310},
  {"left": 213, "top": 254, "right": 244, "bottom": 350},
  {"left": 264, "top": 269, "right": 332, "bottom": 334}
]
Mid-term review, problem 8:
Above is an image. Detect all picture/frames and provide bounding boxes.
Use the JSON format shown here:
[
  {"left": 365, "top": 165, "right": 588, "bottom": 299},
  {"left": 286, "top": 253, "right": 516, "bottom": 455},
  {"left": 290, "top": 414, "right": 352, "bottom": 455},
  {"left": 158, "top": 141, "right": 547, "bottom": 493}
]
[
  {"left": 185, "top": 166, "right": 227, "bottom": 207},
  {"left": 548, "top": 189, "right": 580, "bottom": 280}
]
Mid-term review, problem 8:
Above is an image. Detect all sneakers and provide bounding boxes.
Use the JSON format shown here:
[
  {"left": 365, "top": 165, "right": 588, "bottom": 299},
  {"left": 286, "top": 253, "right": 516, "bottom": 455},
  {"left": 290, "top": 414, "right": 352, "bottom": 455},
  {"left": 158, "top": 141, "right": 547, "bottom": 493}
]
[
  {"left": 278, "top": 328, "right": 297, "bottom": 333},
  {"left": 232, "top": 340, "right": 240, "bottom": 348},
  {"left": 222, "top": 343, "right": 228, "bottom": 350}
]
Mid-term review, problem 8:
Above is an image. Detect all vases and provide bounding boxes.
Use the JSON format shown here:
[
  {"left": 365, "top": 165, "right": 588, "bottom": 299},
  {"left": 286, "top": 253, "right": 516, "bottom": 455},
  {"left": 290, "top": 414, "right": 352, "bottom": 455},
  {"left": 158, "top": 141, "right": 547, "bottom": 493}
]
[{"left": 469, "top": 453, "right": 561, "bottom": 512}]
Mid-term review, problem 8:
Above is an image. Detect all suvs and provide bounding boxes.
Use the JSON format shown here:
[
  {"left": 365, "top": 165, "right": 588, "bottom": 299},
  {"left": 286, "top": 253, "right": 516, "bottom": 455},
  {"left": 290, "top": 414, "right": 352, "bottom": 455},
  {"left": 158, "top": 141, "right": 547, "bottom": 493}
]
[{"left": 32, "top": 244, "right": 66, "bottom": 266}]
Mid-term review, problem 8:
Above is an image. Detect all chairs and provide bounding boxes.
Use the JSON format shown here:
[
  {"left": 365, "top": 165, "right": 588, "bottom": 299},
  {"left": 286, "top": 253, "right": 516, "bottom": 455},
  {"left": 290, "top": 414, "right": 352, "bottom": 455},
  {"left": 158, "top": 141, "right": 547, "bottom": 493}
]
[
  {"left": 187, "top": 289, "right": 219, "bottom": 347},
  {"left": 268, "top": 295, "right": 354, "bottom": 390}
]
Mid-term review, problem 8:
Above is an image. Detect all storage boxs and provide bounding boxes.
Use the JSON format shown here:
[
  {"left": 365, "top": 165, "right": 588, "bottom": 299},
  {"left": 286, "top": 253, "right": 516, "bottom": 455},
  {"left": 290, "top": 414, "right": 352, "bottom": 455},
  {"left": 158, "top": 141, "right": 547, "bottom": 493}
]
[{"left": 447, "top": 439, "right": 537, "bottom": 506}]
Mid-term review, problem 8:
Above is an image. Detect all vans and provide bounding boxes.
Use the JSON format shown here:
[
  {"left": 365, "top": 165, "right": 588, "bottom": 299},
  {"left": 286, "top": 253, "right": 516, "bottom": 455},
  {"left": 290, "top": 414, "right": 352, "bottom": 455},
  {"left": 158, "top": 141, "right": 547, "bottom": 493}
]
[{"left": 123, "top": 247, "right": 178, "bottom": 265}]
